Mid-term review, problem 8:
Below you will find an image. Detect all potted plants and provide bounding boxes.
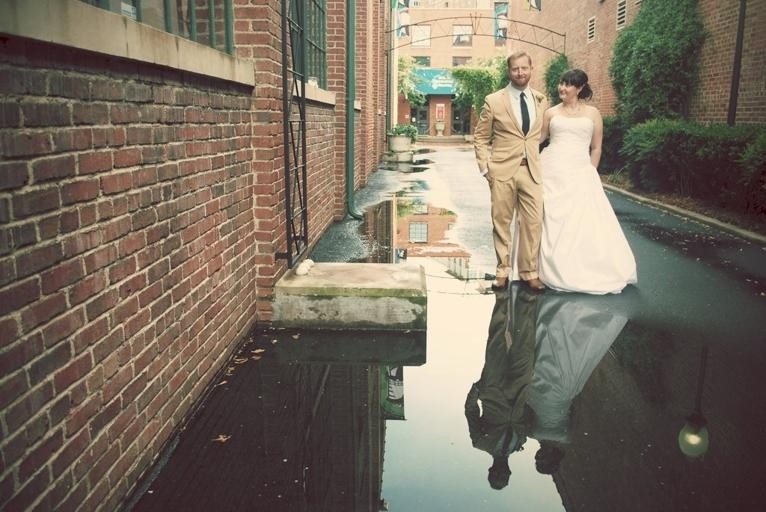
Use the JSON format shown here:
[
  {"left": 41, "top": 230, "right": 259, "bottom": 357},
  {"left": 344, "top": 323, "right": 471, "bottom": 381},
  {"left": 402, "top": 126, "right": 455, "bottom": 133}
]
[{"left": 387, "top": 125, "right": 417, "bottom": 152}]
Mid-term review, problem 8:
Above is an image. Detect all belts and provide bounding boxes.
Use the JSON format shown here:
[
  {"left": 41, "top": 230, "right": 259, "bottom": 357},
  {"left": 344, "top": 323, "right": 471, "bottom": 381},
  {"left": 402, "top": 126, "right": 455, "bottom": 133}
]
[{"left": 520, "top": 160, "right": 529, "bottom": 166}]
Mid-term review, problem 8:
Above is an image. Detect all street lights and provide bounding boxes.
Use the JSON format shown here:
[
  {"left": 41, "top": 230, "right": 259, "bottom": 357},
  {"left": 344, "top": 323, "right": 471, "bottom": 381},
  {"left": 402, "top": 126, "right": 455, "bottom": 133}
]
[
  {"left": 679, "top": 320, "right": 709, "bottom": 458},
  {"left": 393, "top": 7, "right": 409, "bottom": 130}
]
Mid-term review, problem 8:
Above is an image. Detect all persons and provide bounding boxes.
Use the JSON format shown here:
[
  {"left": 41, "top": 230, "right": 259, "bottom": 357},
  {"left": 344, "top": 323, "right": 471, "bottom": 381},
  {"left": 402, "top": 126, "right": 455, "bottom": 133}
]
[
  {"left": 464, "top": 291, "right": 538, "bottom": 490},
  {"left": 505, "top": 293, "right": 633, "bottom": 473},
  {"left": 474, "top": 52, "right": 549, "bottom": 290},
  {"left": 509, "top": 68, "right": 639, "bottom": 296}
]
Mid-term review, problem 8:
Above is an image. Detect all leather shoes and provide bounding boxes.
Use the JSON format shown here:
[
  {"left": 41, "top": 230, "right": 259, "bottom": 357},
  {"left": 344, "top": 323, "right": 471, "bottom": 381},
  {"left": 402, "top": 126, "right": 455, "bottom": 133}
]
[
  {"left": 492, "top": 277, "right": 508, "bottom": 290},
  {"left": 527, "top": 279, "right": 546, "bottom": 291}
]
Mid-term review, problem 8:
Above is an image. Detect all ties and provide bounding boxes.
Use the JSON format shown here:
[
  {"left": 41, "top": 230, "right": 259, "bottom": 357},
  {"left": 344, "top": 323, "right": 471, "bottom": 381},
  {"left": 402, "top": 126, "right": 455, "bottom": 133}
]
[
  {"left": 504, "top": 429, "right": 513, "bottom": 454},
  {"left": 520, "top": 92, "right": 529, "bottom": 135}
]
